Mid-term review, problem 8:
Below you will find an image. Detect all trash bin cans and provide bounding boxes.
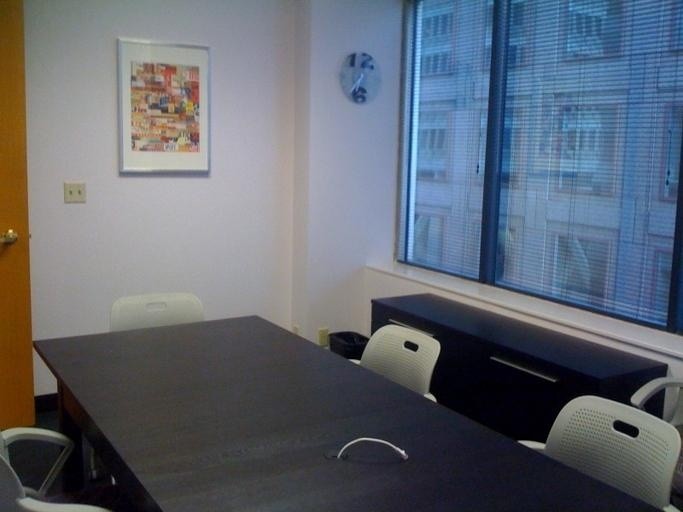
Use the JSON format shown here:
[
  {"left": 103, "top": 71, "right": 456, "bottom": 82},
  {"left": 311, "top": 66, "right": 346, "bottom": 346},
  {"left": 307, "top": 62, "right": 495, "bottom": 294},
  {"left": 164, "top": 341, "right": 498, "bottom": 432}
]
[{"left": 329, "top": 331, "right": 370, "bottom": 364}]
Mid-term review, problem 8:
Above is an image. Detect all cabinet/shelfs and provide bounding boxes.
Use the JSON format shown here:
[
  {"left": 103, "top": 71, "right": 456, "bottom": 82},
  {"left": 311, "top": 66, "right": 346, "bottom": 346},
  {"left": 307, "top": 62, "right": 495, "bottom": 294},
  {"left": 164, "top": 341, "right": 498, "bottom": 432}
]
[{"left": 371, "top": 293, "right": 666, "bottom": 441}]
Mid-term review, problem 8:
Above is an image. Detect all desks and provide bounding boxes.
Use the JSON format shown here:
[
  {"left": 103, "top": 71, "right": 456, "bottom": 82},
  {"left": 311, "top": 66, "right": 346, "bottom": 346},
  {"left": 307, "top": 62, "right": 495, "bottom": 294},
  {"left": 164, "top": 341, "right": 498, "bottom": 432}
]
[{"left": 29, "top": 315, "right": 663, "bottom": 512}]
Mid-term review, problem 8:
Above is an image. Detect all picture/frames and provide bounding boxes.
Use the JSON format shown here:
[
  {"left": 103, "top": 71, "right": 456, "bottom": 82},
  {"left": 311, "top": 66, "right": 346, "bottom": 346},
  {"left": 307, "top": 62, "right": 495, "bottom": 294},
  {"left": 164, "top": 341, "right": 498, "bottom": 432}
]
[{"left": 116, "top": 36, "right": 210, "bottom": 175}]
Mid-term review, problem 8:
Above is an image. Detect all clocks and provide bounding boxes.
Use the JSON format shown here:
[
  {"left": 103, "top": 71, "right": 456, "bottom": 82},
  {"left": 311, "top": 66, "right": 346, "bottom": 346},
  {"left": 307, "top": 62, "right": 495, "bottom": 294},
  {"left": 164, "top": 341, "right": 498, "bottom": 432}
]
[{"left": 338, "top": 52, "right": 382, "bottom": 104}]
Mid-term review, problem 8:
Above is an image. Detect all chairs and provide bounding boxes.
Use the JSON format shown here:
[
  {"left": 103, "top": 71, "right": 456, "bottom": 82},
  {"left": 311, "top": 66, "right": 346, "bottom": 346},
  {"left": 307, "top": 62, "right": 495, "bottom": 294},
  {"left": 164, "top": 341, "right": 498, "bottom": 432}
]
[
  {"left": 0, "top": 427, "right": 112, "bottom": 512},
  {"left": 349, "top": 323, "right": 680, "bottom": 511}
]
